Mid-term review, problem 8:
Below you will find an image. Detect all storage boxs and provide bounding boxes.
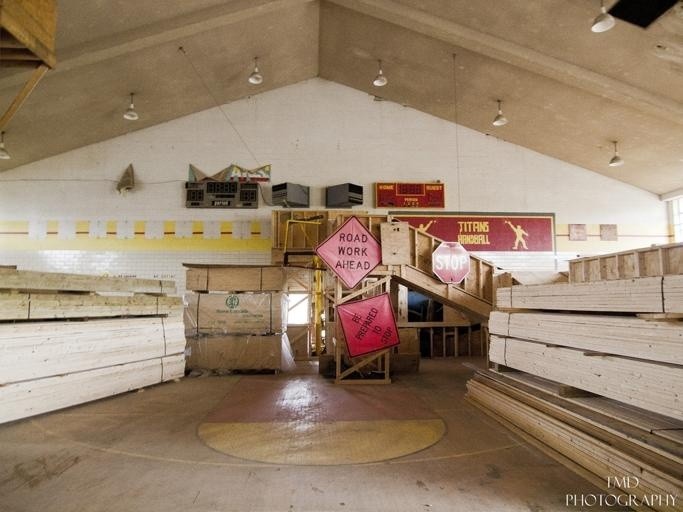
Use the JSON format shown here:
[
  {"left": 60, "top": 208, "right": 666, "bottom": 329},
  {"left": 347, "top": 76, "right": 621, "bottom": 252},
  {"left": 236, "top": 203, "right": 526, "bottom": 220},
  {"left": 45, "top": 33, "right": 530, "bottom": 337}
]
[
  {"left": 325, "top": 326, "right": 421, "bottom": 376},
  {"left": 183, "top": 267, "right": 287, "bottom": 370}
]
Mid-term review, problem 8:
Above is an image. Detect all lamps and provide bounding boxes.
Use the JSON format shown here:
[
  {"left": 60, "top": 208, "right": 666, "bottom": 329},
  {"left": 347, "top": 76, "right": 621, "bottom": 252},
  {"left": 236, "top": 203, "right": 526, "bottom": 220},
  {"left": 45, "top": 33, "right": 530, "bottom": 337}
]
[
  {"left": 0, "top": 132, "right": 11, "bottom": 162},
  {"left": 122, "top": 94, "right": 138, "bottom": 124},
  {"left": 589, "top": 5, "right": 619, "bottom": 38},
  {"left": 490, "top": 99, "right": 509, "bottom": 128},
  {"left": 606, "top": 136, "right": 624, "bottom": 168},
  {"left": 249, "top": 55, "right": 265, "bottom": 84},
  {"left": 372, "top": 61, "right": 387, "bottom": 89}
]
